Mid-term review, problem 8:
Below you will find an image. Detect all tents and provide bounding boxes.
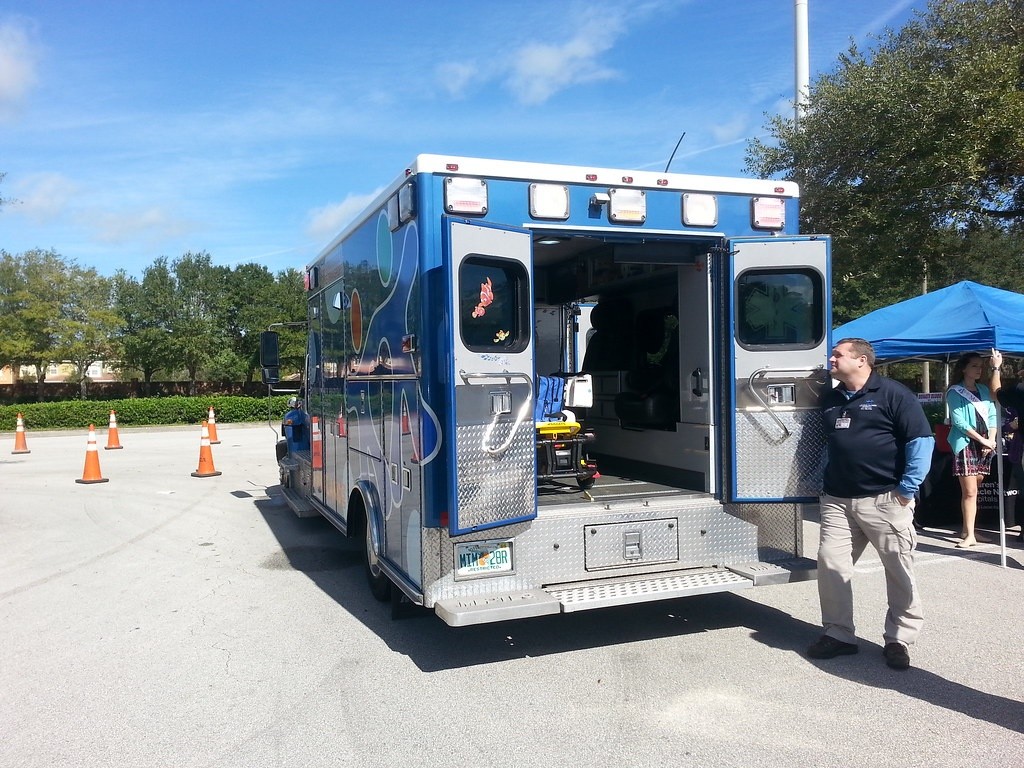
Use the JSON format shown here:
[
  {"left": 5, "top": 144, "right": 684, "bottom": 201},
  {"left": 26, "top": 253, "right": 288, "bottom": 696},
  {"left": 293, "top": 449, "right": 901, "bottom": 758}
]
[{"left": 831, "top": 279, "right": 1024, "bottom": 567}]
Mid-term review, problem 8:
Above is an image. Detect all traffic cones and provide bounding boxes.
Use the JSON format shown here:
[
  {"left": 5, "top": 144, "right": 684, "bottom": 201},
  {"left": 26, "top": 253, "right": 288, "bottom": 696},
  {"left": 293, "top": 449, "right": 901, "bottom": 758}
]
[
  {"left": 191, "top": 421, "right": 222, "bottom": 477},
  {"left": 312, "top": 416, "right": 323, "bottom": 471},
  {"left": 402, "top": 403, "right": 411, "bottom": 435},
  {"left": 207, "top": 404, "right": 221, "bottom": 444},
  {"left": 104, "top": 409, "right": 123, "bottom": 450},
  {"left": 11, "top": 411, "right": 31, "bottom": 454},
  {"left": 336, "top": 411, "right": 346, "bottom": 437},
  {"left": 75, "top": 423, "right": 109, "bottom": 484}
]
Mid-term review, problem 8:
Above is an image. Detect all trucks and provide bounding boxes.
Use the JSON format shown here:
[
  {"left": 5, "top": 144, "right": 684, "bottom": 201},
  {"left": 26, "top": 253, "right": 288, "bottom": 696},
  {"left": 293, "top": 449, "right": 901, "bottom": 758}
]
[{"left": 259, "top": 132, "right": 834, "bottom": 628}]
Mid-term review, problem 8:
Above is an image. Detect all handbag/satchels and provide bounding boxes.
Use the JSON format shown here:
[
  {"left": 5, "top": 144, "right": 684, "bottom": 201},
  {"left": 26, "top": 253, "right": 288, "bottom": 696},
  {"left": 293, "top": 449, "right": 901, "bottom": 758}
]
[{"left": 935, "top": 412, "right": 952, "bottom": 452}]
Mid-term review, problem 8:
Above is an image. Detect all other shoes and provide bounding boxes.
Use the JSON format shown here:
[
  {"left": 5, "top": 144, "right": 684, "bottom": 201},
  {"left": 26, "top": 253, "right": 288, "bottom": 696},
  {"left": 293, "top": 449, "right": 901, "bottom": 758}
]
[
  {"left": 806, "top": 634, "right": 858, "bottom": 659},
  {"left": 913, "top": 517, "right": 925, "bottom": 529},
  {"left": 956, "top": 537, "right": 977, "bottom": 548},
  {"left": 929, "top": 519, "right": 936, "bottom": 528},
  {"left": 960, "top": 531, "right": 982, "bottom": 539},
  {"left": 882, "top": 642, "right": 910, "bottom": 670}
]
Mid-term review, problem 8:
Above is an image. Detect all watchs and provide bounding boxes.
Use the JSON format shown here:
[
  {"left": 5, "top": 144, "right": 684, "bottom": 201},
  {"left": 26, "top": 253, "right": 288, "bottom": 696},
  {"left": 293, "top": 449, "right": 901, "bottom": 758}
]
[{"left": 991, "top": 366, "right": 1000, "bottom": 372}]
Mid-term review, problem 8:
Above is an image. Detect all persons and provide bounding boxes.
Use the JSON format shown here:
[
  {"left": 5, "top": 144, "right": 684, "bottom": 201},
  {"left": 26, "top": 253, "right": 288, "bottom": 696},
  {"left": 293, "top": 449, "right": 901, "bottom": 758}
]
[
  {"left": 944, "top": 353, "right": 1000, "bottom": 547},
  {"left": 808, "top": 337, "right": 935, "bottom": 668},
  {"left": 988, "top": 347, "right": 1024, "bottom": 544}
]
